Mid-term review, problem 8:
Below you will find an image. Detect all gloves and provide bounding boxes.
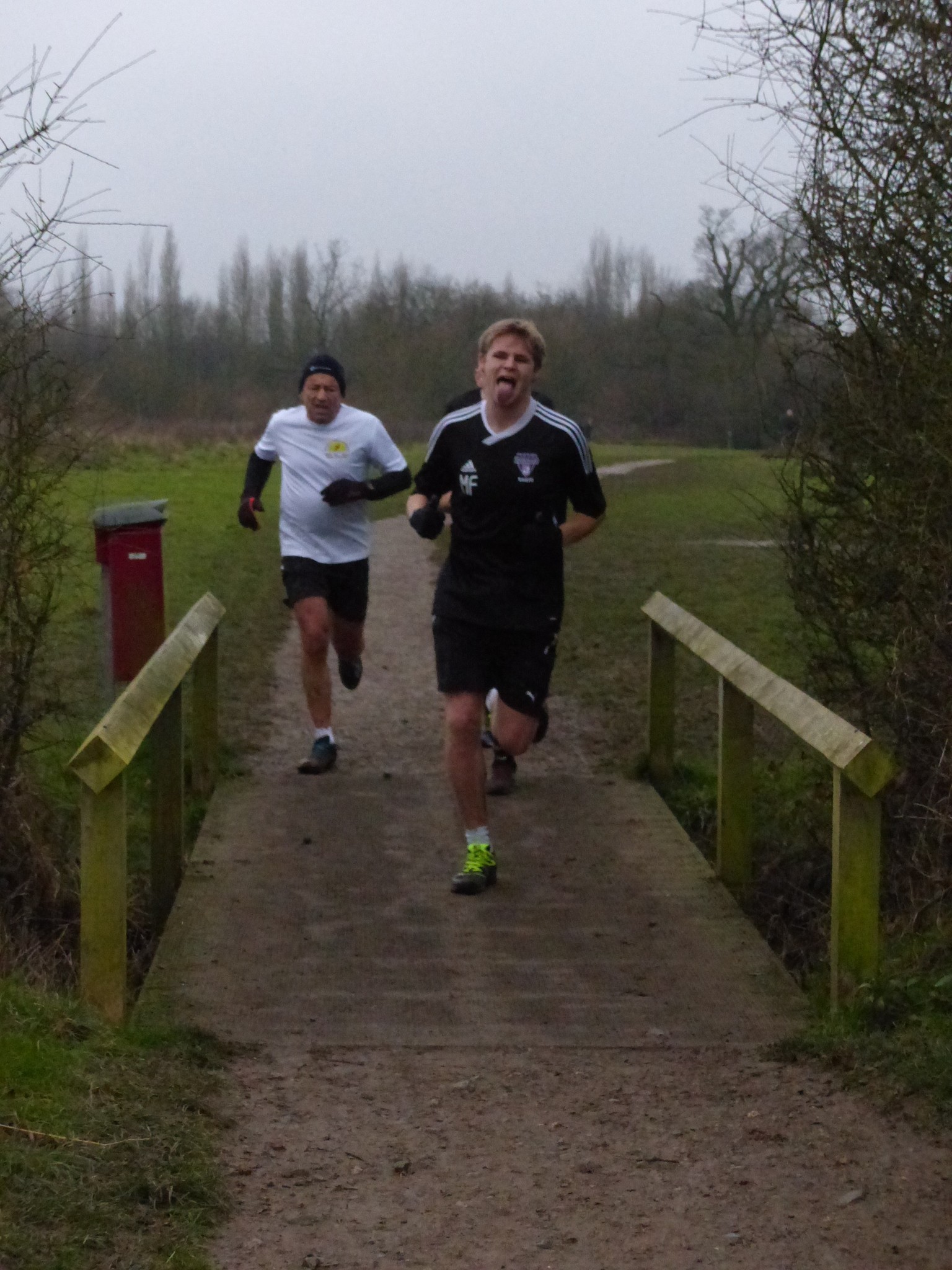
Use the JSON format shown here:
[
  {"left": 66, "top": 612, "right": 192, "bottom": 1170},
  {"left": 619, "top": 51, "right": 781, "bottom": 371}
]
[
  {"left": 410, "top": 495, "right": 446, "bottom": 541},
  {"left": 319, "top": 479, "right": 370, "bottom": 506},
  {"left": 521, "top": 519, "right": 564, "bottom": 559},
  {"left": 237, "top": 496, "right": 265, "bottom": 531}
]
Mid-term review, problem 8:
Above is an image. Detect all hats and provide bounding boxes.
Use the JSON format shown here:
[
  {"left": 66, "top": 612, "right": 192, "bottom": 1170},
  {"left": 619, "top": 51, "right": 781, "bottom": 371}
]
[{"left": 299, "top": 354, "right": 348, "bottom": 397}]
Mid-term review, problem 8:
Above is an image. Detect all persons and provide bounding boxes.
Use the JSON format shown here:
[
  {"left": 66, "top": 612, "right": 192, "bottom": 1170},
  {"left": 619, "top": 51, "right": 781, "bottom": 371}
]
[
  {"left": 407, "top": 317, "right": 608, "bottom": 897},
  {"left": 437, "top": 387, "right": 566, "bottom": 745},
  {"left": 236, "top": 356, "right": 415, "bottom": 775}
]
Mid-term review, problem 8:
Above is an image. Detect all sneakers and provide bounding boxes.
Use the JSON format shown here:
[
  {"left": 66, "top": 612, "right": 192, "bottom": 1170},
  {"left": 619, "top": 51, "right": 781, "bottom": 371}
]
[
  {"left": 452, "top": 844, "right": 498, "bottom": 892},
  {"left": 481, "top": 728, "right": 504, "bottom": 755},
  {"left": 337, "top": 652, "right": 365, "bottom": 693},
  {"left": 490, "top": 745, "right": 516, "bottom": 795},
  {"left": 298, "top": 739, "right": 338, "bottom": 774}
]
